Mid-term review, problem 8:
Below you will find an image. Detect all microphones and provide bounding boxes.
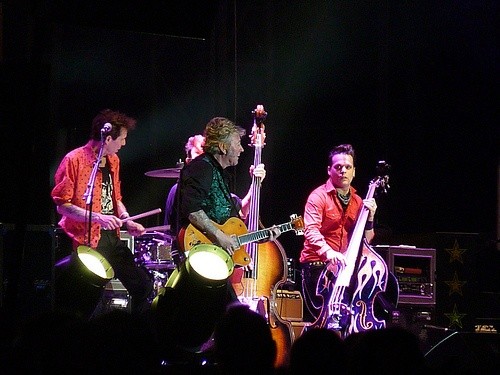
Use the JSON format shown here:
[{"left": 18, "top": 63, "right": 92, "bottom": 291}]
[{"left": 101, "top": 123, "right": 112, "bottom": 132}]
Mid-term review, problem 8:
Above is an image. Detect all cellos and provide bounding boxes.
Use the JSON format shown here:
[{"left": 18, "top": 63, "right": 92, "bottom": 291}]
[
  {"left": 299, "top": 175, "right": 401, "bottom": 339},
  {"left": 232, "top": 104, "right": 291, "bottom": 368}
]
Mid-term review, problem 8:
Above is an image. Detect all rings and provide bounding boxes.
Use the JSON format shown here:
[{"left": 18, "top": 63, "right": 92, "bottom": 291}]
[{"left": 372, "top": 205, "right": 374, "bottom": 207}]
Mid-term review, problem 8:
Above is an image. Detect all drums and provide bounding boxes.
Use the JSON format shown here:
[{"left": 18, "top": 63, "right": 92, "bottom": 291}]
[{"left": 132, "top": 232, "right": 175, "bottom": 270}]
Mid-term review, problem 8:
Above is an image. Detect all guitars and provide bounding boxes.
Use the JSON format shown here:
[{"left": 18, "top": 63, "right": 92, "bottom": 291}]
[{"left": 174, "top": 215, "right": 308, "bottom": 269}]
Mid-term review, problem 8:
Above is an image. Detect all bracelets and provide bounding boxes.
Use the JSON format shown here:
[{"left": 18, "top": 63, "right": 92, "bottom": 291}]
[{"left": 365, "top": 220, "right": 374, "bottom": 231}]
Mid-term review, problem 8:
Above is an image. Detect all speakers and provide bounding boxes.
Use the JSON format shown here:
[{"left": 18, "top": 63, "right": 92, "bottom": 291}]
[{"left": 274, "top": 289, "right": 304, "bottom": 321}]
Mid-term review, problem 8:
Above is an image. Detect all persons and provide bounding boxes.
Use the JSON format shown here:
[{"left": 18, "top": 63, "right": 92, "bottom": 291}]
[
  {"left": 0, "top": 287, "right": 500, "bottom": 375},
  {"left": 176, "top": 117, "right": 282, "bottom": 308},
  {"left": 54, "top": 108, "right": 147, "bottom": 325},
  {"left": 298, "top": 145, "right": 378, "bottom": 325},
  {"left": 163, "top": 134, "right": 266, "bottom": 269}
]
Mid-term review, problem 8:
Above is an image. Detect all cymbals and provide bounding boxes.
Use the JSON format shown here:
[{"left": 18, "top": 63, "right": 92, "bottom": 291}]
[{"left": 144, "top": 167, "right": 183, "bottom": 179}]
